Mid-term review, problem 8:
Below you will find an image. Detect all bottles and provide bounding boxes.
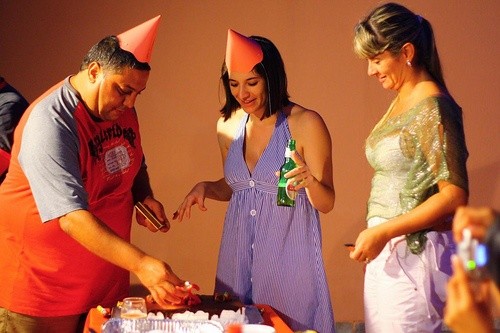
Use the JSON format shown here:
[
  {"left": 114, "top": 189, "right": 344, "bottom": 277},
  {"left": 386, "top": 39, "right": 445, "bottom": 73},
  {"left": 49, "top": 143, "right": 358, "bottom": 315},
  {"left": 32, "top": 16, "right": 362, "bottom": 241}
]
[{"left": 276, "top": 140, "right": 298, "bottom": 207}]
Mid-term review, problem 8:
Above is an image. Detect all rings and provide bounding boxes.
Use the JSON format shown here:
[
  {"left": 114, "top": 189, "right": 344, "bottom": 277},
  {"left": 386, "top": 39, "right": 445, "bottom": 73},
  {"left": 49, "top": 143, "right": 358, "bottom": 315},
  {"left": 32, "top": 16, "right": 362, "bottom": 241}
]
[{"left": 365, "top": 258, "right": 371, "bottom": 263}]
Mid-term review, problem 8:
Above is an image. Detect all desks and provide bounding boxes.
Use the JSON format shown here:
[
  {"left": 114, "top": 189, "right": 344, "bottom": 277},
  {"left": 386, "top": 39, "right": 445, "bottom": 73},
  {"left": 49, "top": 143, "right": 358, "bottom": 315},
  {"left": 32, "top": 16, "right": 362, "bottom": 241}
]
[{"left": 83, "top": 304, "right": 294, "bottom": 333}]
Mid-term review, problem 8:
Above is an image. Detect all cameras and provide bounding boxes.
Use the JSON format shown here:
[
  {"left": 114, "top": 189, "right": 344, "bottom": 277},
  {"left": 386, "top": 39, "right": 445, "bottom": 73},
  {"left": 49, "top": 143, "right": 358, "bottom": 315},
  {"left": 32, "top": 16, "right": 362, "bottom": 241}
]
[{"left": 458, "top": 228, "right": 487, "bottom": 278}]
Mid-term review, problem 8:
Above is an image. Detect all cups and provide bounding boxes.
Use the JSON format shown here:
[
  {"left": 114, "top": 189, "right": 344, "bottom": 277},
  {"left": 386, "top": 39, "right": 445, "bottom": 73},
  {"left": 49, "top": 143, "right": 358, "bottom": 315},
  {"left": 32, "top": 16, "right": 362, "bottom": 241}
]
[{"left": 121, "top": 298, "right": 147, "bottom": 319}]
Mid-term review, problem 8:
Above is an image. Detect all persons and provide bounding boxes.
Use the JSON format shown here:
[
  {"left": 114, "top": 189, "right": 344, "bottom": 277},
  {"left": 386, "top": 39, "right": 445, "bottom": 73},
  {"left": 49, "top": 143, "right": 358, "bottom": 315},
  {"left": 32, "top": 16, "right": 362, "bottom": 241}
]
[
  {"left": 0, "top": 15, "right": 188, "bottom": 333},
  {"left": 172, "top": 28, "right": 335, "bottom": 333},
  {"left": 442, "top": 206, "right": 500, "bottom": 333},
  {"left": 350, "top": 2, "right": 470, "bottom": 333}
]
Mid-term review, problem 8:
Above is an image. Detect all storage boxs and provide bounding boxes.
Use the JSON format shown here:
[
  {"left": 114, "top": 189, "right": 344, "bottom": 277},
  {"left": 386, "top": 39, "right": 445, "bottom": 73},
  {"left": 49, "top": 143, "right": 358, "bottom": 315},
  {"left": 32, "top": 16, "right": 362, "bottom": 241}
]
[{"left": 102, "top": 319, "right": 224, "bottom": 333}]
